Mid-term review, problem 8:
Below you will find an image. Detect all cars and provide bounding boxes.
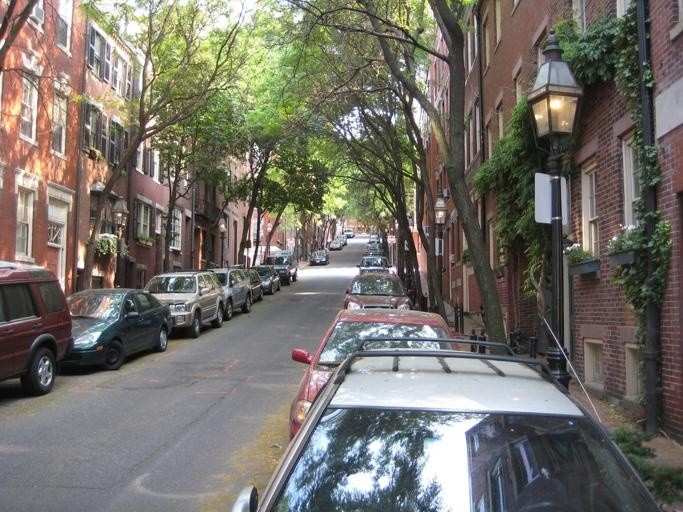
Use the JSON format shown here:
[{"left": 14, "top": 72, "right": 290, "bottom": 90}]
[
  {"left": 229, "top": 338, "right": 665, "bottom": 512},
  {"left": 344, "top": 229, "right": 410, "bottom": 311},
  {"left": 290, "top": 308, "right": 464, "bottom": 444},
  {"left": 60, "top": 256, "right": 300, "bottom": 372},
  {"left": 309, "top": 234, "right": 347, "bottom": 265}
]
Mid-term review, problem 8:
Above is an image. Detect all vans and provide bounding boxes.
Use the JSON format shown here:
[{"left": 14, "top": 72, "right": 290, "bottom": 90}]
[{"left": 1, "top": 267, "right": 70, "bottom": 395}]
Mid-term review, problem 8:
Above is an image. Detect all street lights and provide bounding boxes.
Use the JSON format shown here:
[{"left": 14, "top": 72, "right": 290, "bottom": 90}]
[
  {"left": 526, "top": 27, "right": 583, "bottom": 394},
  {"left": 267, "top": 222, "right": 273, "bottom": 256},
  {"left": 434, "top": 198, "right": 448, "bottom": 313},
  {"left": 218, "top": 223, "right": 228, "bottom": 268},
  {"left": 111, "top": 194, "right": 131, "bottom": 287}
]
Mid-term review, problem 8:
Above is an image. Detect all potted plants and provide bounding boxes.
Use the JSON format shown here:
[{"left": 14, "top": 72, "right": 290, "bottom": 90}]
[
  {"left": 606, "top": 223, "right": 638, "bottom": 268},
  {"left": 138, "top": 236, "right": 154, "bottom": 248},
  {"left": 563, "top": 243, "right": 599, "bottom": 275}
]
